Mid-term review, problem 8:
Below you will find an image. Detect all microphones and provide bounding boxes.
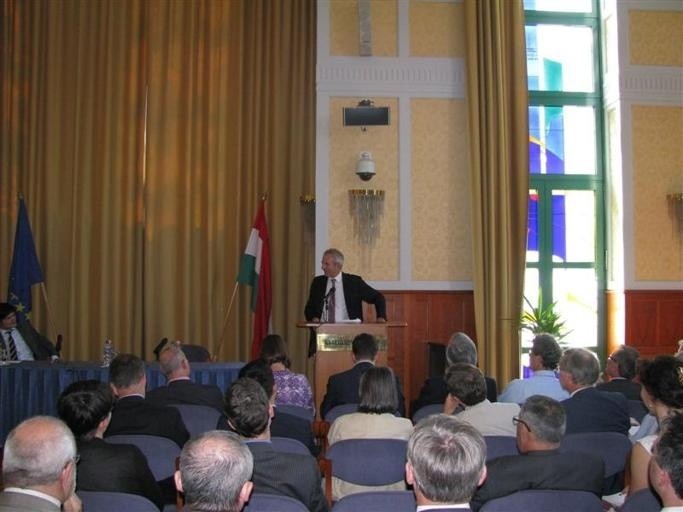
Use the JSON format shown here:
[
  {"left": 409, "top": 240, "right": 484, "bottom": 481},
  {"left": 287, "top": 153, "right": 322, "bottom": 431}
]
[
  {"left": 153, "top": 338, "right": 167, "bottom": 361},
  {"left": 56, "top": 334, "right": 62, "bottom": 361},
  {"left": 321, "top": 288, "right": 336, "bottom": 303}
]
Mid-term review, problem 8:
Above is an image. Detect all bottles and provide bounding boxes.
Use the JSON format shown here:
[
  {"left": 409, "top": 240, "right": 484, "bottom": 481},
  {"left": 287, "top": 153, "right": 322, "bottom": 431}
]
[{"left": 103, "top": 340, "right": 113, "bottom": 366}]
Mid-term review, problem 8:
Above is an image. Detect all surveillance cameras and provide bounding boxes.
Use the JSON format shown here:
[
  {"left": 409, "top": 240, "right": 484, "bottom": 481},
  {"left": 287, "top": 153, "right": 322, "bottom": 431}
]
[{"left": 357, "top": 152, "right": 376, "bottom": 182}]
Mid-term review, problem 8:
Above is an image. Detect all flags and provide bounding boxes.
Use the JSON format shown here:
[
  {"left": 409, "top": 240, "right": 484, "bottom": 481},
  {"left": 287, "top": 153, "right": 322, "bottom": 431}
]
[
  {"left": 234, "top": 198, "right": 273, "bottom": 362},
  {"left": 6, "top": 198, "right": 44, "bottom": 323}
]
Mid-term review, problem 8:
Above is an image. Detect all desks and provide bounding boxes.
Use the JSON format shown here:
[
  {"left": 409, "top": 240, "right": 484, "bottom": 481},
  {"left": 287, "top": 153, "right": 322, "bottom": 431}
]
[{"left": 0, "top": 361, "right": 250, "bottom": 452}]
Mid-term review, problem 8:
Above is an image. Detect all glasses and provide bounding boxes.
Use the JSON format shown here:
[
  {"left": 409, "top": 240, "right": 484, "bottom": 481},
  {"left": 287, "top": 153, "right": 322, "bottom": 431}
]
[
  {"left": 71, "top": 455, "right": 80, "bottom": 462},
  {"left": 529, "top": 349, "right": 535, "bottom": 356},
  {"left": 608, "top": 355, "right": 617, "bottom": 363},
  {"left": 511, "top": 415, "right": 531, "bottom": 433}
]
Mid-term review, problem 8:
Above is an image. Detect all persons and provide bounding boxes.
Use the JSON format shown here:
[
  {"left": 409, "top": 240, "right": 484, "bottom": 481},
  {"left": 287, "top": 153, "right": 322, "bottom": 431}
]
[
  {"left": 0, "top": 415, "right": 83, "bottom": 512},
  {"left": 173, "top": 430, "right": 254, "bottom": 512},
  {"left": 320, "top": 367, "right": 414, "bottom": 502},
  {"left": 405, "top": 413, "right": 488, "bottom": 512},
  {"left": 145, "top": 332, "right": 497, "bottom": 411},
  {"left": 224, "top": 377, "right": 329, "bottom": 512},
  {"left": 496, "top": 332, "right": 683, "bottom": 512},
  {"left": 102, "top": 352, "right": 190, "bottom": 449},
  {"left": 442, "top": 363, "right": 522, "bottom": 438},
  {"left": 469, "top": 394, "right": 602, "bottom": 512},
  {"left": 216, "top": 358, "right": 315, "bottom": 454},
  {"left": 304, "top": 248, "right": 388, "bottom": 358},
  {"left": 0, "top": 302, "right": 60, "bottom": 366},
  {"left": 56, "top": 379, "right": 165, "bottom": 512}
]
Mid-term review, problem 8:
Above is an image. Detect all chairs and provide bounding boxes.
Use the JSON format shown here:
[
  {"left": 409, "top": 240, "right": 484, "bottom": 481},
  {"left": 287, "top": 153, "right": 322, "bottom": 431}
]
[{"left": 158, "top": 344, "right": 210, "bottom": 361}]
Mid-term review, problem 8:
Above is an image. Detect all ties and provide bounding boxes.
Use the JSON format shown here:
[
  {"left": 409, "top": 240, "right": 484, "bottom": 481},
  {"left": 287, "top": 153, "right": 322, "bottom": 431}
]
[
  {"left": 4, "top": 329, "right": 18, "bottom": 362},
  {"left": 328, "top": 279, "right": 336, "bottom": 323}
]
